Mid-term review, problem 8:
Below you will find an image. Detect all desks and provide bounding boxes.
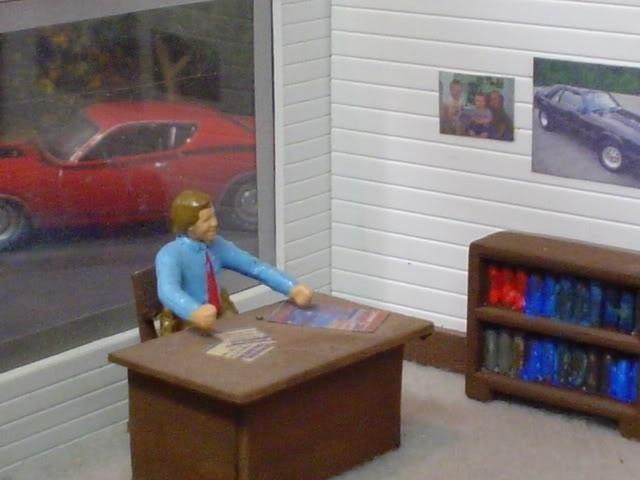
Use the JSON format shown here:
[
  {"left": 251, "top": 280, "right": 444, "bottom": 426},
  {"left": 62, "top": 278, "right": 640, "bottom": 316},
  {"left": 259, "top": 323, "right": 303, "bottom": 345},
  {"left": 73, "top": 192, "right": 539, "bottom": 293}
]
[{"left": 108, "top": 292, "right": 434, "bottom": 479}]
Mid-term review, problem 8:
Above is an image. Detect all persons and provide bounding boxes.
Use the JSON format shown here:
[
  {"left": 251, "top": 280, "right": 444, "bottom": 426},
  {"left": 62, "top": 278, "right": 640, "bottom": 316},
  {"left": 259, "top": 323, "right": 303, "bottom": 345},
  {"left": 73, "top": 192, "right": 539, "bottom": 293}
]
[
  {"left": 440, "top": 78, "right": 513, "bottom": 141},
  {"left": 155, "top": 189, "right": 313, "bottom": 337}
]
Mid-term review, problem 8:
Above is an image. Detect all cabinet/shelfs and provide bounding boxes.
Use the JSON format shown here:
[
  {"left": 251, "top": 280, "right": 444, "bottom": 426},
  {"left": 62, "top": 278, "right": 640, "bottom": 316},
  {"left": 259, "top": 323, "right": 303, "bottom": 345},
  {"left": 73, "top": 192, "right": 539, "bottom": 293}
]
[{"left": 463, "top": 229, "right": 639, "bottom": 440}]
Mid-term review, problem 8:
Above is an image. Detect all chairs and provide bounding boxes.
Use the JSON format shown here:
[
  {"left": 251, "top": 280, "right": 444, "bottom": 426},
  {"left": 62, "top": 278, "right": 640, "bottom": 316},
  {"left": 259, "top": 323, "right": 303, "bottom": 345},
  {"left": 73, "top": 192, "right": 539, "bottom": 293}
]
[{"left": 130, "top": 266, "right": 157, "bottom": 343}]
